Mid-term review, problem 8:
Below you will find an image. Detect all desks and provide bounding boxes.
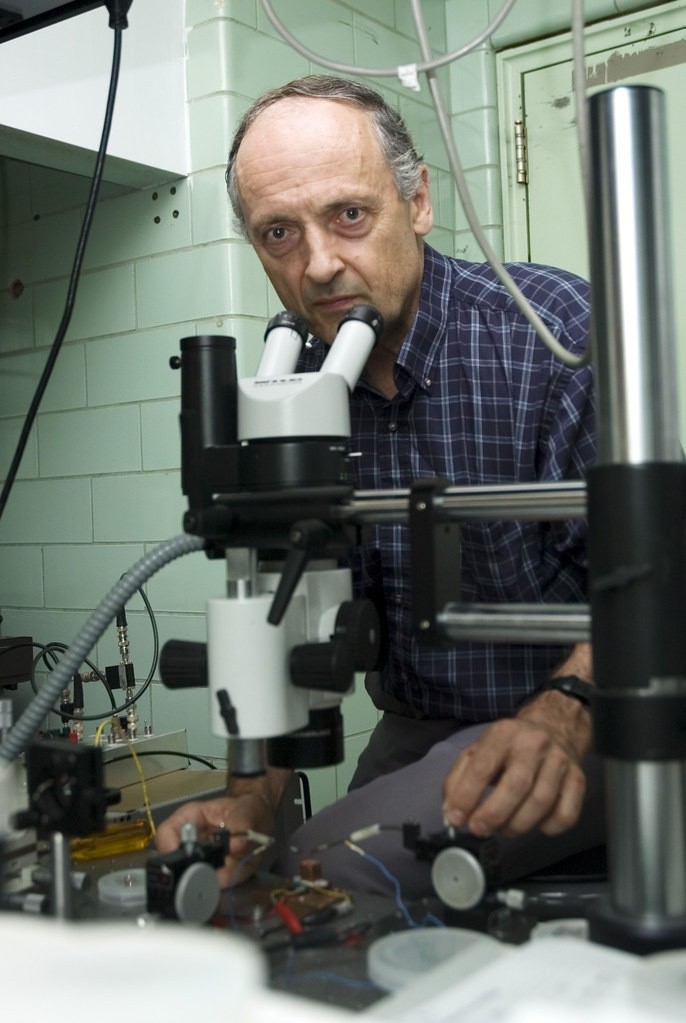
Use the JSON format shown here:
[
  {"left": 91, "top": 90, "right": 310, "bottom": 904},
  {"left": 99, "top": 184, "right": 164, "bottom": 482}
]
[{"left": 0, "top": 764, "right": 420, "bottom": 1011}]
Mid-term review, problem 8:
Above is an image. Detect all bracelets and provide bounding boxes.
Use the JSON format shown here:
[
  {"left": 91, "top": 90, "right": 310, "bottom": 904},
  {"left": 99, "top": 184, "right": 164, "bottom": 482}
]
[{"left": 538, "top": 675, "right": 598, "bottom": 716}]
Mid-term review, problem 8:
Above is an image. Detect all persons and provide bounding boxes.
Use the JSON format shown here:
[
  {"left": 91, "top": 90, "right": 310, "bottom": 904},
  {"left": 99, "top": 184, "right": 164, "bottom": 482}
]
[{"left": 149, "top": 77, "right": 598, "bottom": 916}]
